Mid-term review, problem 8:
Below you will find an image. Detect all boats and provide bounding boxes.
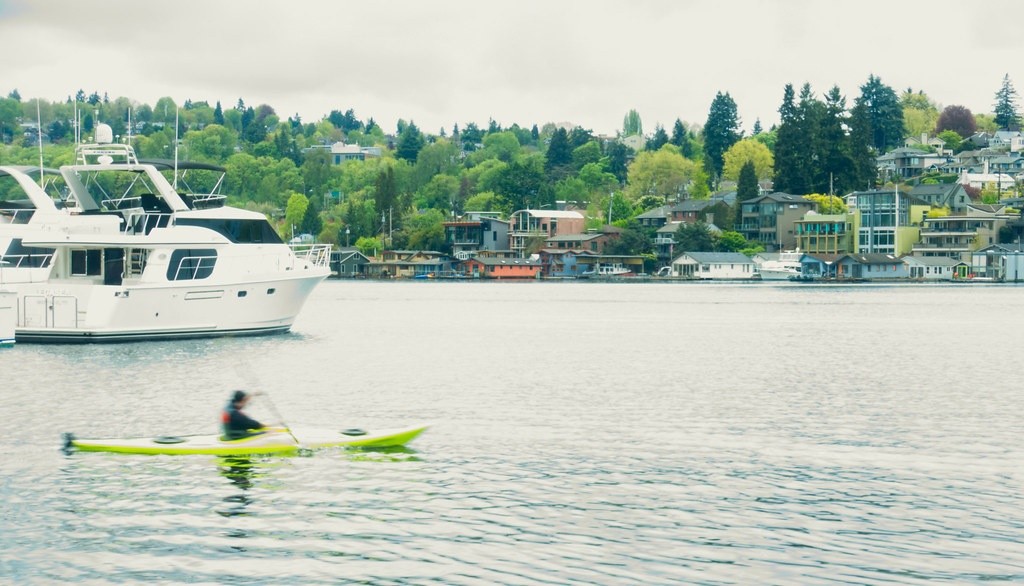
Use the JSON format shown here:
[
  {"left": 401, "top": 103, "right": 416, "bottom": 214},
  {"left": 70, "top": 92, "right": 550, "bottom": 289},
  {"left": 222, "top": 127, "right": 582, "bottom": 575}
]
[
  {"left": 65, "top": 423, "right": 433, "bottom": 457},
  {"left": 0, "top": 97, "right": 335, "bottom": 345}
]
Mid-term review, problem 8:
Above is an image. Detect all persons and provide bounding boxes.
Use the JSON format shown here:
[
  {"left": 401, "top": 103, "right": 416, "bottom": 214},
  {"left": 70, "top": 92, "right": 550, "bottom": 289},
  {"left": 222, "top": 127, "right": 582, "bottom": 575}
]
[{"left": 222, "top": 390, "right": 282, "bottom": 441}]
